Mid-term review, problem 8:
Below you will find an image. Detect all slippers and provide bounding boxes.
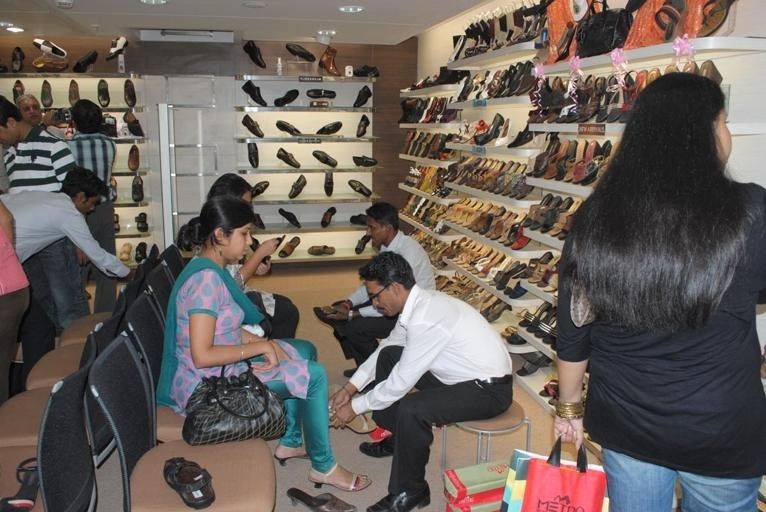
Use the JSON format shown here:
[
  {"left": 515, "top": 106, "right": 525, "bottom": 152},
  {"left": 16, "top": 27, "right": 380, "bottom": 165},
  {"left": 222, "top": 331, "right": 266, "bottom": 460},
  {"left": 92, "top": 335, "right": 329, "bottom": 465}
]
[
  {"left": 518, "top": 355, "right": 546, "bottom": 375},
  {"left": 287, "top": 484, "right": 355, "bottom": 512},
  {"left": 696, "top": 0, "right": 736, "bottom": 37},
  {"left": 395, "top": 0, "right": 575, "bottom": 344},
  {"left": 113, "top": 214, "right": 120, "bottom": 233},
  {"left": 655, "top": 0, "right": 688, "bottom": 43}
]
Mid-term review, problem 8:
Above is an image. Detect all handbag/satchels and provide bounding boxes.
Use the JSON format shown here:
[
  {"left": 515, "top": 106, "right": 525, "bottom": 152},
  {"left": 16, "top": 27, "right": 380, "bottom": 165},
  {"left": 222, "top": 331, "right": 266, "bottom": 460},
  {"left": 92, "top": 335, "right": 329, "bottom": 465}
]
[
  {"left": 499, "top": 432, "right": 608, "bottom": 512},
  {"left": 182, "top": 358, "right": 287, "bottom": 446},
  {"left": 575, "top": 0, "right": 633, "bottom": 58}
]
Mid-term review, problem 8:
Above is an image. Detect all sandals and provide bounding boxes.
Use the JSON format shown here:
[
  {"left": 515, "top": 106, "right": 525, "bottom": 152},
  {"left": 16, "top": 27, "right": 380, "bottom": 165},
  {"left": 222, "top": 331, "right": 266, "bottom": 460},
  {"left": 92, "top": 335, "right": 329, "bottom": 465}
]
[
  {"left": 306, "top": 463, "right": 371, "bottom": 487},
  {"left": 328, "top": 406, "right": 377, "bottom": 434},
  {"left": 0, "top": 458, "right": 41, "bottom": 512},
  {"left": 577, "top": 56, "right": 725, "bottom": 125},
  {"left": 164, "top": 457, "right": 215, "bottom": 509}
]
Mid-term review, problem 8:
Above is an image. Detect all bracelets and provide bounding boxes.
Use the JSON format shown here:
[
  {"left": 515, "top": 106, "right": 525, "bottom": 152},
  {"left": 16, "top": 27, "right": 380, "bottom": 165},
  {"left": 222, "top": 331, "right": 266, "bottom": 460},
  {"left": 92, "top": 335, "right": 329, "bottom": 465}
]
[
  {"left": 249, "top": 334, "right": 258, "bottom": 343},
  {"left": 347, "top": 310, "right": 354, "bottom": 321},
  {"left": 555, "top": 401, "right": 583, "bottom": 419},
  {"left": 341, "top": 303, "right": 350, "bottom": 310},
  {"left": 343, "top": 387, "right": 351, "bottom": 400},
  {"left": 240, "top": 344, "right": 244, "bottom": 360}
]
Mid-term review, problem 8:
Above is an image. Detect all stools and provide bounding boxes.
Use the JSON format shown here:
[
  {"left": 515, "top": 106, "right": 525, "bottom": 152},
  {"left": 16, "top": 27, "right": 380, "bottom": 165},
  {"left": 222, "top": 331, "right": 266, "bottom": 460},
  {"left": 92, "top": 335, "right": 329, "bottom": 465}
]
[{"left": 441, "top": 401, "right": 532, "bottom": 478}]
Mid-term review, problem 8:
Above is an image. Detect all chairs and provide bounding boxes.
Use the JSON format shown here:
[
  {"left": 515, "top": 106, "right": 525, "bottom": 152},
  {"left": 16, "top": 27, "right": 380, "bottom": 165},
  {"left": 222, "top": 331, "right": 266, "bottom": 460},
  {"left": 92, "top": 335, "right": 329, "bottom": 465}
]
[{"left": 0, "top": 243, "right": 275, "bottom": 512}]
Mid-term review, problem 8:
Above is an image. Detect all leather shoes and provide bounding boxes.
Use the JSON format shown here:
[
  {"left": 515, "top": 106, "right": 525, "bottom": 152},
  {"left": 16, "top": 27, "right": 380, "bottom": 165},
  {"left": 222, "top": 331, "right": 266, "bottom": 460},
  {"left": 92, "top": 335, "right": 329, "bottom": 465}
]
[
  {"left": 243, "top": 41, "right": 380, "bottom": 265},
  {"left": 344, "top": 368, "right": 357, "bottom": 377},
  {"left": 367, "top": 480, "right": 430, "bottom": 512},
  {"left": 120, "top": 79, "right": 147, "bottom": 267},
  {"left": 0, "top": 36, "right": 129, "bottom": 73},
  {"left": 13, "top": 79, "right": 109, "bottom": 107},
  {"left": 360, "top": 434, "right": 396, "bottom": 457},
  {"left": 110, "top": 178, "right": 117, "bottom": 202}
]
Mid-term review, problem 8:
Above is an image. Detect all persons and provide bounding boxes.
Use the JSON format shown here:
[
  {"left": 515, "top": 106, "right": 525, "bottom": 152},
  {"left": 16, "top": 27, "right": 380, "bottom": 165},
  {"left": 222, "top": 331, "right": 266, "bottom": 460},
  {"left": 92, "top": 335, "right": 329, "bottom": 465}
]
[
  {"left": 553, "top": 73, "right": 765, "bottom": 512},
  {"left": 328, "top": 250, "right": 513, "bottom": 512},
  {"left": 207, "top": 172, "right": 300, "bottom": 341},
  {"left": 173, "top": 216, "right": 203, "bottom": 260},
  {"left": 0, "top": 94, "right": 138, "bottom": 405},
  {"left": 321, "top": 201, "right": 437, "bottom": 377},
  {"left": 155, "top": 195, "right": 369, "bottom": 492}
]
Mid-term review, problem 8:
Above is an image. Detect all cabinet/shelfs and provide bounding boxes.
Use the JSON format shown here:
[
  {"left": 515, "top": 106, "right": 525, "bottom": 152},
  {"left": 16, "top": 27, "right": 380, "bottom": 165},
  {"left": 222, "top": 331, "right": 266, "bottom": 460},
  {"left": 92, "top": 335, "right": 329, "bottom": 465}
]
[
  {"left": 236, "top": 71, "right": 379, "bottom": 264},
  {"left": 514, "top": 35, "right": 765, "bottom": 496},
  {"left": 399, "top": 84, "right": 466, "bottom": 292},
  {"left": 441, "top": 44, "right": 542, "bottom": 354},
  {"left": 0, "top": 72, "right": 151, "bottom": 269}
]
